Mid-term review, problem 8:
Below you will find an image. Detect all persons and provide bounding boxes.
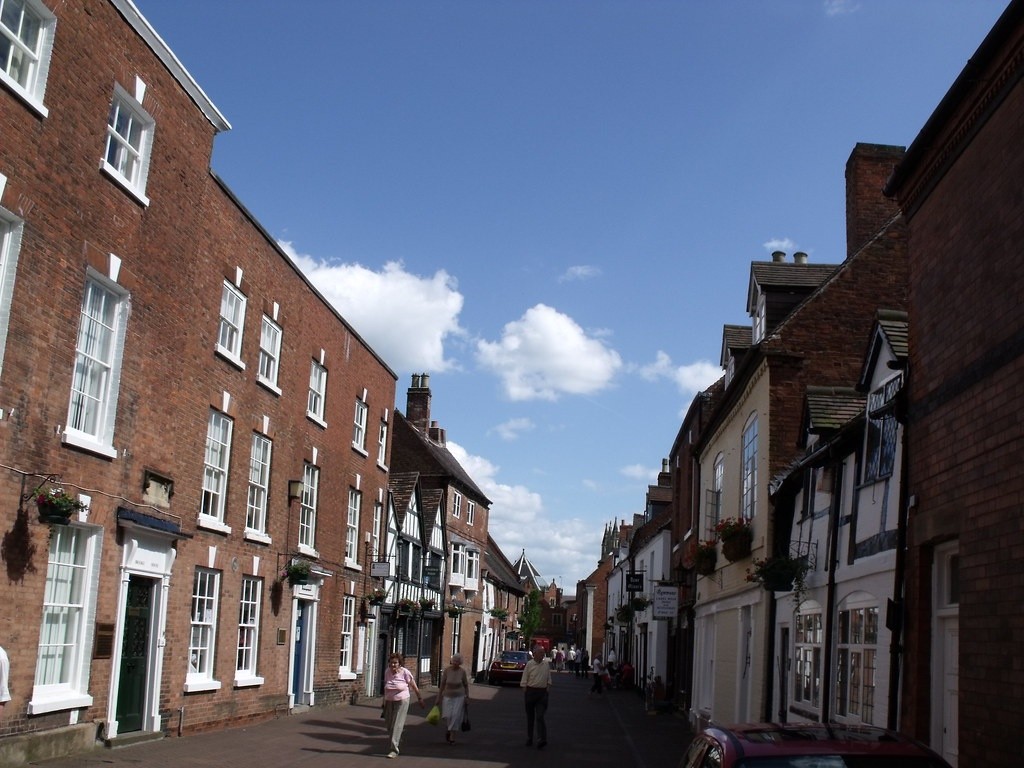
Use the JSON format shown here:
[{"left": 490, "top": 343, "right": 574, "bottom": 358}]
[
  {"left": 383, "top": 653, "right": 425, "bottom": 759},
  {"left": 521, "top": 645, "right": 552, "bottom": 748},
  {"left": 435, "top": 654, "right": 471, "bottom": 745},
  {"left": 518, "top": 642, "right": 635, "bottom": 696}
]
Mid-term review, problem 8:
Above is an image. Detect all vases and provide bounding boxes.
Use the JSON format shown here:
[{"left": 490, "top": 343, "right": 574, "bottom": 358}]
[
  {"left": 38, "top": 503, "right": 73, "bottom": 518},
  {"left": 449, "top": 613, "right": 458, "bottom": 618},
  {"left": 374, "top": 597, "right": 383, "bottom": 602},
  {"left": 762, "top": 571, "right": 795, "bottom": 591},
  {"left": 693, "top": 550, "right": 717, "bottom": 576},
  {"left": 421, "top": 604, "right": 432, "bottom": 612},
  {"left": 722, "top": 537, "right": 750, "bottom": 562},
  {"left": 290, "top": 573, "right": 308, "bottom": 581},
  {"left": 401, "top": 605, "right": 410, "bottom": 612}
]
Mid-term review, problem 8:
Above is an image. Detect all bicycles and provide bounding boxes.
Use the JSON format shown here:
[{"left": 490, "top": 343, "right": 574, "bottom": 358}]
[{"left": 644, "top": 665, "right": 661, "bottom": 711}]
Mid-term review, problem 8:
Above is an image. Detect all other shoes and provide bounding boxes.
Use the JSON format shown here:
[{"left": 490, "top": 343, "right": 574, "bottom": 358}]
[
  {"left": 535, "top": 740, "right": 545, "bottom": 750},
  {"left": 447, "top": 738, "right": 456, "bottom": 746},
  {"left": 388, "top": 751, "right": 397, "bottom": 758},
  {"left": 445, "top": 731, "right": 450, "bottom": 741},
  {"left": 526, "top": 739, "right": 534, "bottom": 747}
]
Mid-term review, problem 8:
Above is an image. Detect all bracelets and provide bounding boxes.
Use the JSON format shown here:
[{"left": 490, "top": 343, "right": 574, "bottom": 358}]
[{"left": 418, "top": 698, "right": 422, "bottom": 700}]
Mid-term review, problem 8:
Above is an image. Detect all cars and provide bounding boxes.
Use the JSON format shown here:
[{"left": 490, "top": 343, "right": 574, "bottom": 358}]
[
  {"left": 679, "top": 723, "right": 952, "bottom": 768},
  {"left": 488, "top": 651, "right": 533, "bottom": 684}
]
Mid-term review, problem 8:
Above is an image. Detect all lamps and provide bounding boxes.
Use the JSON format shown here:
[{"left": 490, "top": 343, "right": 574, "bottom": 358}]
[
  {"left": 289, "top": 479, "right": 305, "bottom": 506},
  {"left": 673, "top": 554, "right": 697, "bottom": 589}
]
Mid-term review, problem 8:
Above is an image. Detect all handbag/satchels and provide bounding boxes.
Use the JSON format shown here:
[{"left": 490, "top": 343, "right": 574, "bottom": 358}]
[
  {"left": 425, "top": 705, "right": 440, "bottom": 726},
  {"left": 460, "top": 702, "right": 470, "bottom": 732}
]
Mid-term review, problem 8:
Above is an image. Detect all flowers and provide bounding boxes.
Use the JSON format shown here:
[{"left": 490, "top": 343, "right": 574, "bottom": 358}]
[
  {"left": 26, "top": 486, "right": 90, "bottom": 514},
  {"left": 398, "top": 598, "right": 419, "bottom": 613},
  {"left": 691, "top": 539, "right": 718, "bottom": 550},
  {"left": 444, "top": 606, "right": 467, "bottom": 614},
  {"left": 418, "top": 597, "right": 435, "bottom": 606},
  {"left": 279, "top": 558, "right": 318, "bottom": 584},
  {"left": 744, "top": 551, "right": 817, "bottom": 617},
  {"left": 361, "top": 586, "right": 387, "bottom": 601},
  {"left": 709, "top": 514, "right": 753, "bottom": 539}
]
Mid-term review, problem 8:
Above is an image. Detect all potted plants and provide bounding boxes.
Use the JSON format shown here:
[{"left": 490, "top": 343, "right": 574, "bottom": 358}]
[
  {"left": 630, "top": 597, "right": 645, "bottom": 611},
  {"left": 637, "top": 623, "right": 647, "bottom": 633}
]
